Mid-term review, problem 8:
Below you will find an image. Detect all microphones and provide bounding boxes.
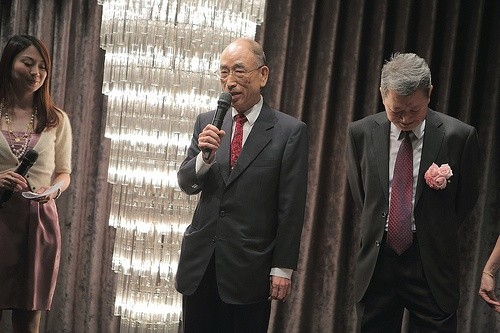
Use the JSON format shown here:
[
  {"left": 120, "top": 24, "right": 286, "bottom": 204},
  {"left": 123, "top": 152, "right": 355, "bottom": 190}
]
[
  {"left": 204, "top": 92, "right": 232, "bottom": 160},
  {"left": 0, "top": 149, "right": 39, "bottom": 209}
]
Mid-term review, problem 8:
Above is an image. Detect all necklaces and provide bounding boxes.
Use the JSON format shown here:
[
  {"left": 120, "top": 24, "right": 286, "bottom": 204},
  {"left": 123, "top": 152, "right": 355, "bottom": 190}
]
[{"left": 0, "top": 95, "right": 38, "bottom": 160}]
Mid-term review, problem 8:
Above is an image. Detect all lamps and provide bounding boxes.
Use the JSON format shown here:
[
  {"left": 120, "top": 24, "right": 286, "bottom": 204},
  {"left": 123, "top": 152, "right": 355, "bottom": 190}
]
[{"left": 97, "top": 0, "right": 265, "bottom": 333}]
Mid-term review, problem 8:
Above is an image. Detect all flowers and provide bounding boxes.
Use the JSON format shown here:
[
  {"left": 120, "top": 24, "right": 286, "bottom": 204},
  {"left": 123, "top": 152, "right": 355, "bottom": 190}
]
[{"left": 424, "top": 162, "right": 453, "bottom": 190}]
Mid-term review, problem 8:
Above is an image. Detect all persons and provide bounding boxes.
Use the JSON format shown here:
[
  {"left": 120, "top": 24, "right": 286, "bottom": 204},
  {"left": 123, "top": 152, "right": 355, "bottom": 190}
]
[
  {"left": 478, "top": 233, "right": 500, "bottom": 313},
  {"left": 173, "top": 36, "right": 308, "bottom": 333},
  {"left": 0, "top": 34, "right": 73, "bottom": 333},
  {"left": 344, "top": 51, "right": 482, "bottom": 333}
]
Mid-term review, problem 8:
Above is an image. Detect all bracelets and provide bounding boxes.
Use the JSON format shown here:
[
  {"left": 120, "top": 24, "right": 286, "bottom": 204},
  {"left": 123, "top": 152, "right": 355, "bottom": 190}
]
[
  {"left": 52, "top": 185, "right": 62, "bottom": 199},
  {"left": 482, "top": 271, "right": 496, "bottom": 280}
]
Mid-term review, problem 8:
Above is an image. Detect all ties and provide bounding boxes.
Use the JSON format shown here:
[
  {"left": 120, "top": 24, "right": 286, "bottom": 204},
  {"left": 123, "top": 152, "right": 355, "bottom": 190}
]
[
  {"left": 387, "top": 130, "right": 413, "bottom": 257},
  {"left": 230, "top": 113, "right": 248, "bottom": 172}
]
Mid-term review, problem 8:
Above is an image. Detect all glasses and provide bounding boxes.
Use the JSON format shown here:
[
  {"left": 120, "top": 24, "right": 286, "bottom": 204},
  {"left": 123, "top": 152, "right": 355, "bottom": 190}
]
[{"left": 214, "top": 65, "right": 265, "bottom": 80}]
